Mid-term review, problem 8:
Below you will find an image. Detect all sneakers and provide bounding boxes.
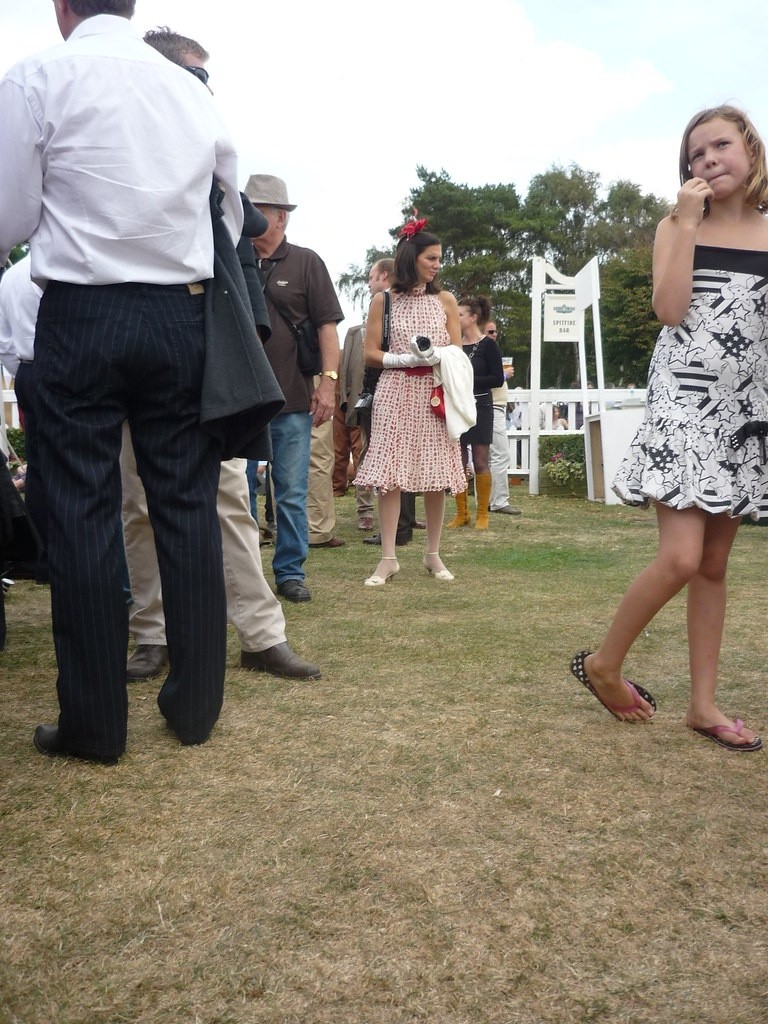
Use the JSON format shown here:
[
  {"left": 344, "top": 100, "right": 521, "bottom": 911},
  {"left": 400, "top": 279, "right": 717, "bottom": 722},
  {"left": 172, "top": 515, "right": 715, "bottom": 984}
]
[{"left": 277, "top": 579, "right": 311, "bottom": 602}]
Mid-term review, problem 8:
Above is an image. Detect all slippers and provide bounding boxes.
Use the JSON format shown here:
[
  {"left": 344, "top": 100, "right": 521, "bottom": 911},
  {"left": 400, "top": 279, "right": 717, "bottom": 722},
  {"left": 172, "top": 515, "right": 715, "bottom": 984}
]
[
  {"left": 569, "top": 650, "right": 656, "bottom": 722},
  {"left": 691, "top": 718, "right": 763, "bottom": 752}
]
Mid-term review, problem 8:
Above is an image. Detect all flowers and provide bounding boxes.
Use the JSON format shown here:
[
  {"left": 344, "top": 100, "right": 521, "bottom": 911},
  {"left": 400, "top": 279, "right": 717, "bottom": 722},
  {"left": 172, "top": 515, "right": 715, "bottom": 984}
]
[
  {"left": 542, "top": 453, "right": 584, "bottom": 486},
  {"left": 395, "top": 207, "right": 430, "bottom": 240}
]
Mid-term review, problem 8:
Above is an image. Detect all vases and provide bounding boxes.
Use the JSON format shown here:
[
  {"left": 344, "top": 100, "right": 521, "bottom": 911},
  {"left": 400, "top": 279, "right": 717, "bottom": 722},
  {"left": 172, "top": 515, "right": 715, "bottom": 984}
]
[{"left": 538, "top": 466, "right": 588, "bottom": 496}]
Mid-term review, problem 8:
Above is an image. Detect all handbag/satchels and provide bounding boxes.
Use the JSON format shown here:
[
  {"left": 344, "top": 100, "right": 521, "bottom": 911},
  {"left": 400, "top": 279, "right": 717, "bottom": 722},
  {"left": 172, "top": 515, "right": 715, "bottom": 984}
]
[
  {"left": 296, "top": 324, "right": 322, "bottom": 375},
  {"left": 430, "top": 386, "right": 445, "bottom": 419}
]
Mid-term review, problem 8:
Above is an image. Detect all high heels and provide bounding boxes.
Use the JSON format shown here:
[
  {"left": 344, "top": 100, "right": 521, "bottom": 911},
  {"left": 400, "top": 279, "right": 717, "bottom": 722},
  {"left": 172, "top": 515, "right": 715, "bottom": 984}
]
[
  {"left": 364, "top": 557, "right": 399, "bottom": 587},
  {"left": 425, "top": 552, "right": 454, "bottom": 580}
]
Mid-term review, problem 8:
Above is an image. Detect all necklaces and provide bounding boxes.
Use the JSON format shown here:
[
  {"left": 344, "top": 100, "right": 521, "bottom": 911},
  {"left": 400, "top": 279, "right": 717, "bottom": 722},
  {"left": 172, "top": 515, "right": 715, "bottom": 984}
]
[{"left": 468, "top": 334, "right": 481, "bottom": 362}]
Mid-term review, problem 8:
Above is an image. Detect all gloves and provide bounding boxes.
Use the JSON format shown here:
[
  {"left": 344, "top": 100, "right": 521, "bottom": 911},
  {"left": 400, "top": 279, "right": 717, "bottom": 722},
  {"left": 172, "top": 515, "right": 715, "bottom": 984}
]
[
  {"left": 410, "top": 335, "right": 433, "bottom": 357},
  {"left": 382, "top": 345, "right": 440, "bottom": 368}
]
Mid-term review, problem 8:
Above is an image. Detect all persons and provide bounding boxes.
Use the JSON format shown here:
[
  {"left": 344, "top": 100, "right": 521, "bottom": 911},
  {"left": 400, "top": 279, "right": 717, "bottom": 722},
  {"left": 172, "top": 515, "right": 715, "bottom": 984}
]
[
  {"left": 0, "top": 0, "right": 245, "bottom": 767},
  {"left": 0, "top": 25, "right": 638, "bottom": 681},
  {"left": 567, "top": 99, "right": 768, "bottom": 755}
]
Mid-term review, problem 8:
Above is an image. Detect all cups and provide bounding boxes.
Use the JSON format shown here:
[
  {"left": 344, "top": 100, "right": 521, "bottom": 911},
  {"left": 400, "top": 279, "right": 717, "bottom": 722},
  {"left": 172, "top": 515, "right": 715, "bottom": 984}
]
[{"left": 502, "top": 357, "right": 513, "bottom": 370}]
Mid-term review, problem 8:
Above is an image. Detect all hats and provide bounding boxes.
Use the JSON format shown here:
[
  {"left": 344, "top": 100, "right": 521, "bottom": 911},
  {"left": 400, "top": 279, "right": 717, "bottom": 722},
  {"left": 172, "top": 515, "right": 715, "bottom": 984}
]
[{"left": 243, "top": 174, "right": 297, "bottom": 212}]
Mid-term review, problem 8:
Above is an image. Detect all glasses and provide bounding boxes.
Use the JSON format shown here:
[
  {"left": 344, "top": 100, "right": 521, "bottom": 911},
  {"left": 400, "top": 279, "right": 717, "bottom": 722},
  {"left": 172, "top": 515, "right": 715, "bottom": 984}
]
[
  {"left": 488, "top": 330, "right": 497, "bottom": 334},
  {"left": 184, "top": 66, "right": 209, "bottom": 84}
]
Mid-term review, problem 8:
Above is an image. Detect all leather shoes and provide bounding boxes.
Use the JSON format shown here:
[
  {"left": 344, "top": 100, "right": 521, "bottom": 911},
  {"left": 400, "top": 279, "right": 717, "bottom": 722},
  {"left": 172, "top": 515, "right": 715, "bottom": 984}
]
[
  {"left": 127, "top": 645, "right": 168, "bottom": 682},
  {"left": 241, "top": 641, "right": 321, "bottom": 682},
  {"left": 34, "top": 725, "right": 58, "bottom": 757},
  {"left": 358, "top": 517, "right": 373, "bottom": 530},
  {"left": 493, "top": 505, "right": 521, "bottom": 514}
]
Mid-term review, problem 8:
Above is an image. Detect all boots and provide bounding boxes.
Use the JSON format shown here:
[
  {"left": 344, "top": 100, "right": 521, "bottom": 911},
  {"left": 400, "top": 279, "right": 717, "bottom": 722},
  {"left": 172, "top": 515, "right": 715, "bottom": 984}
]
[
  {"left": 475, "top": 472, "right": 491, "bottom": 529},
  {"left": 445, "top": 474, "right": 471, "bottom": 527}
]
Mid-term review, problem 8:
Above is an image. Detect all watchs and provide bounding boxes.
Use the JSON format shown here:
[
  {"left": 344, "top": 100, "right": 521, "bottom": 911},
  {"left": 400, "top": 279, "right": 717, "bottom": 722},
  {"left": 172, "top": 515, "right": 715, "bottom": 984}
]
[{"left": 319, "top": 369, "right": 341, "bottom": 381}]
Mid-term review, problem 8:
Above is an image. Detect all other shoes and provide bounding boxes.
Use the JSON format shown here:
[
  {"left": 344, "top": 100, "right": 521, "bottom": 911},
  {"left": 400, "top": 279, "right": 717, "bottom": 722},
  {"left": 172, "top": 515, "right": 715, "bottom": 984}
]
[
  {"left": 309, "top": 537, "right": 345, "bottom": 547},
  {"left": 413, "top": 524, "right": 426, "bottom": 529},
  {"left": 363, "top": 533, "right": 381, "bottom": 544}
]
